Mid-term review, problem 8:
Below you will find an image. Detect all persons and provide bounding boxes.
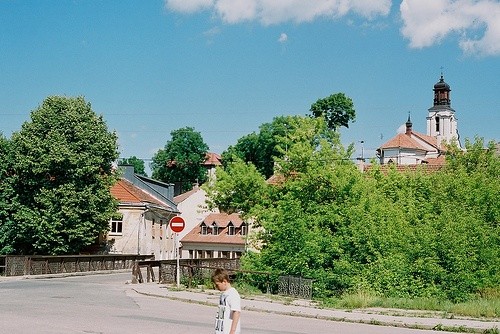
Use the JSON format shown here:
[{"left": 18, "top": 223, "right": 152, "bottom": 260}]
[{"left": 211, "top": 267, "right": 242, "bottom": 334}]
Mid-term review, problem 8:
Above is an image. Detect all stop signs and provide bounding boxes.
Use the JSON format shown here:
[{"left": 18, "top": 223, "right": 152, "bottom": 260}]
[{"left": 169, "top": 216, "right": 186, "bottom": 233}]
